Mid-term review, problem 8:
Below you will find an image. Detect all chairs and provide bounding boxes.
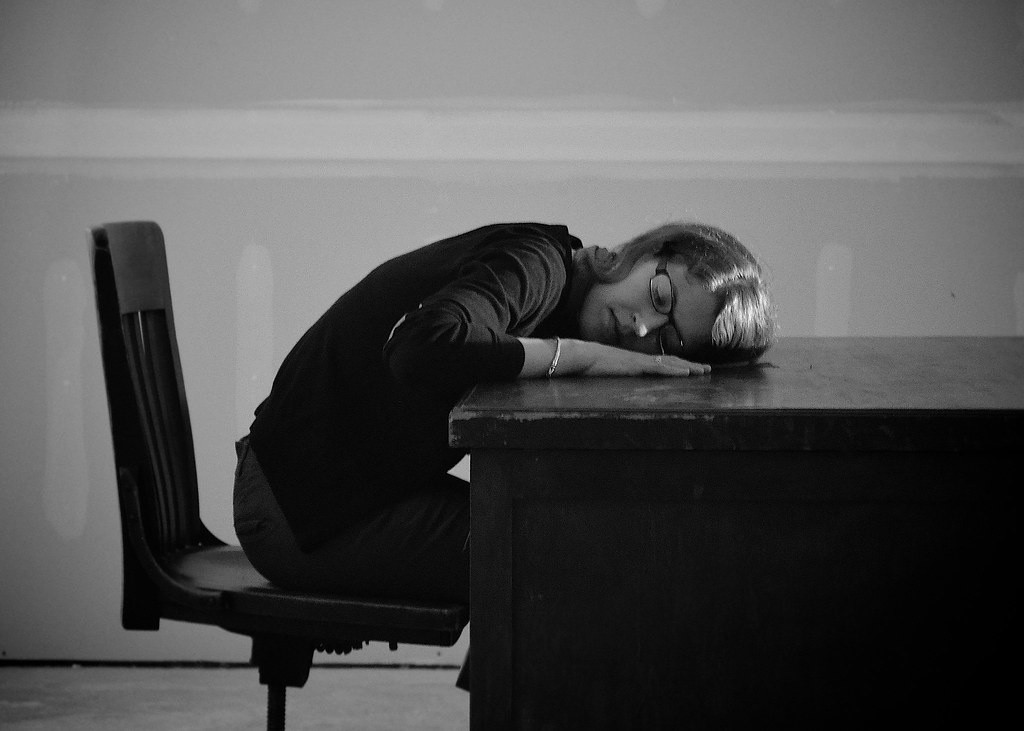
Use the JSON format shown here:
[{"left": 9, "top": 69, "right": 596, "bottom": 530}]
[{"left": 88, "top": 219, "right": 466, "bottom": 731}]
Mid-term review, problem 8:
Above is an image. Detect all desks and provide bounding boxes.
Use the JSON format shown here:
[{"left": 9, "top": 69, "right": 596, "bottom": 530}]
[{"left": 449, "top": 334, "right": 1024, "bottom": 731}]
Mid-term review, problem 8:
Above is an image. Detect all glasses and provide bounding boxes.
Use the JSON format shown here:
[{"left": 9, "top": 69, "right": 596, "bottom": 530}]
[{"left": 648, "top": 251, "right": 684, "bottom": 357}]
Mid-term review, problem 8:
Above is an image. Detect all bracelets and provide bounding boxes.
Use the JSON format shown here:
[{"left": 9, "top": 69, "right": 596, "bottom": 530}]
[{"left": 546, "top": 337, "right": 562, "bottom": 377}]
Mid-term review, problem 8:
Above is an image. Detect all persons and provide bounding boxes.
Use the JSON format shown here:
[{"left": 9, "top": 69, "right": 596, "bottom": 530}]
[{"left": 234, "top": 220, "right": 775, "bottom": 607}]
